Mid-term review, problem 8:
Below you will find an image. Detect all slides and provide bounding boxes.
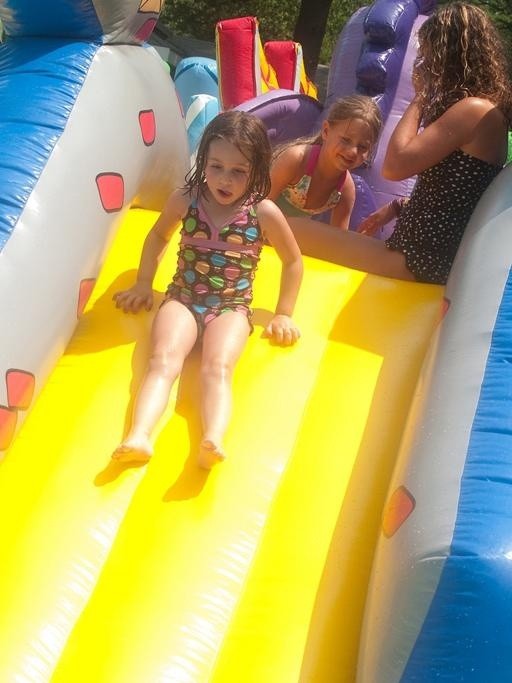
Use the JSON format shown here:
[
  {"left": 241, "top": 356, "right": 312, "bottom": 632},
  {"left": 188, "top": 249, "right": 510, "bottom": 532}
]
[{"left": 0, "top": 207, "right": 509, "bottom": 680}]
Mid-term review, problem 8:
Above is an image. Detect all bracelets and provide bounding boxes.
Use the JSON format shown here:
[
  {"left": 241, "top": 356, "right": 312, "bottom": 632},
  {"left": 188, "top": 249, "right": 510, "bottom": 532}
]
[{"left": 391, "top": 197, "right": 401, "bottom": 218}]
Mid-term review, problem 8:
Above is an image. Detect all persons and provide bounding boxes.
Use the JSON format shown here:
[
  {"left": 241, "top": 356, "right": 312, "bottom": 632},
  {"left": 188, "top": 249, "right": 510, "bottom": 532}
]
[
  {"left": 265, "top": 93, "right": 384, "bottom": 232},
  {"left": 111, "top": 108, "right": 306, "bottom": 465},
  {"left": 356, "top": 0, "right": 510, "bottom": 285}
]
